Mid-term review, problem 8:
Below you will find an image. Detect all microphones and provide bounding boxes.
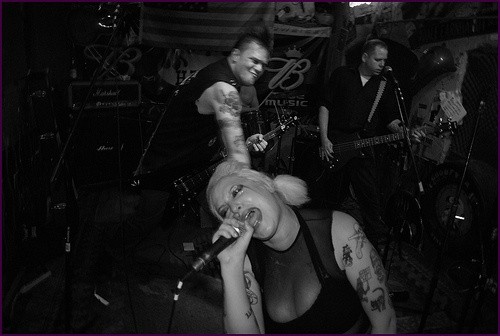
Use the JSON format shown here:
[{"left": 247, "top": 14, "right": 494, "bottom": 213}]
[
  {"left": 177, "top": 225, "right": 243, "bottom": 284},
  {"left": 385, "top": 66, "right": 398, "bottom": 83}
]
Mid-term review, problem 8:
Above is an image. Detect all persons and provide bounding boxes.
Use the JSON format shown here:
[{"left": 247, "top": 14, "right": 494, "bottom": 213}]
[
  {"left": 205, "top": 160, "right": 398, "bottom": 336},
  {"left": 291, "top": 37, "right": 427, "bottom": 277},
  {"left": 94, "top": 32, "right": 272, "bottom": 278}
]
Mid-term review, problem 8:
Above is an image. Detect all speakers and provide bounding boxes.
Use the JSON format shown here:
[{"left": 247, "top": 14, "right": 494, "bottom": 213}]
[
  {"left": 66, "top": 107, "right": 143, "bottom": 190},
  {"left": 291, "top": 128, "right": 345, "bottom": 210}
]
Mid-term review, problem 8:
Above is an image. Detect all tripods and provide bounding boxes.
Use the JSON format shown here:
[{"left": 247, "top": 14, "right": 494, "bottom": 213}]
[
  {"left": 382, "top": 84, "right": 425, "bottom": 281},
  {"left": 262, "top": 110, "right": 307, "bottom": 178}
]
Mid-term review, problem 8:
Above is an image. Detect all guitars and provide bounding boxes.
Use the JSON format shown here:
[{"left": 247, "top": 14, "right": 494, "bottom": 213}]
[
  {"left": 321, "top": 118, "right": 459, "bottom": 177},
  {"left": 166, "top": 113, "right": 305, "bottom": 206}
]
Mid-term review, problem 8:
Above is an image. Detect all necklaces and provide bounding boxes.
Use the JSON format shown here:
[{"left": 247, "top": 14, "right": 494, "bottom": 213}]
[{"left": 359, "top": 65, "right": 374, "bottom": 81}]
